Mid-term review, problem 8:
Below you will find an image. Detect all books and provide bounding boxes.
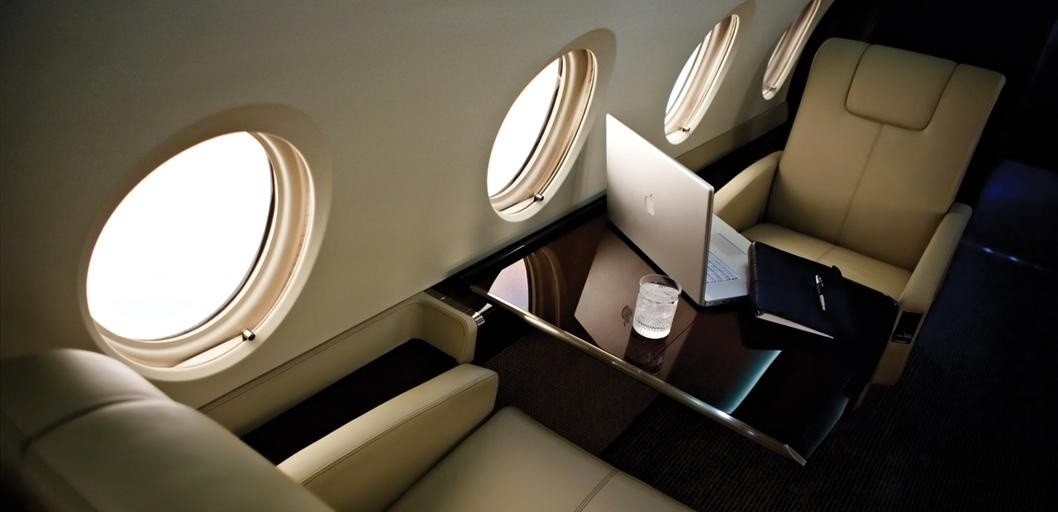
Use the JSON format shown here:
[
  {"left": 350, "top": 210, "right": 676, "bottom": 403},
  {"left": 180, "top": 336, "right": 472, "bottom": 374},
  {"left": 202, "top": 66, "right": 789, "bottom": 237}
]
[{"left": 747, "top": 240, "right": 837, "bottom": 339}]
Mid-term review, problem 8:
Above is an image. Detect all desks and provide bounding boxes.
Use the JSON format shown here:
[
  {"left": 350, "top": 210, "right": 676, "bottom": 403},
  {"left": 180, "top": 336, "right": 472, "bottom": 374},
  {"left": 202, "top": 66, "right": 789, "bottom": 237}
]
[{"left": 460, "top": 227, "right": 902, "bottom": 484}]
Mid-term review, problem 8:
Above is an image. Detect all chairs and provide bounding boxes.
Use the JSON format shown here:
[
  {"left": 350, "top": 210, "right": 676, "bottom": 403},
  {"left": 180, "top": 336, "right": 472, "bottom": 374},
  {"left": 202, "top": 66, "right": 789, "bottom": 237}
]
[
  {"left": 2, "top": 321, "right": 706, "bottom": 512},
  {"left": 693, "top": 28, "right": 1018, "bottom": 399}
]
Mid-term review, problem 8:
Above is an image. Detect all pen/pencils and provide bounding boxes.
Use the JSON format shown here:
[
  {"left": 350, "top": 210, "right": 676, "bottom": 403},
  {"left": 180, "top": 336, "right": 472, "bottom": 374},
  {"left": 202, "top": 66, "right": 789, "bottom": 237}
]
[{"left": 815, "top": 275, "right": 826, "bottom": 311}]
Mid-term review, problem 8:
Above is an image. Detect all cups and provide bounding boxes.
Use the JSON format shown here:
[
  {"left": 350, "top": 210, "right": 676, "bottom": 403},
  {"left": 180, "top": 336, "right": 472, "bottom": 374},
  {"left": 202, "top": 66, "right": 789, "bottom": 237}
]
[
  {"left": 632, "top": 273, "right": 682, "bottom": 343},
  {"left": 624, "top": 330, "right": 666, "bottom": 376}
]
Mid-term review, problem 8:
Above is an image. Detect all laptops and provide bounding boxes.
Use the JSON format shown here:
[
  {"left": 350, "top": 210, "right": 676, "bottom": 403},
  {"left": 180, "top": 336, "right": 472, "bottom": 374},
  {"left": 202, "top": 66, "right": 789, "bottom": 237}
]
[
  {"left": 572, "top": 231, "right": 699, "bottom": 382},
  {"left": 604, "top": 110, "right": 753, "bottom": 308}
]
